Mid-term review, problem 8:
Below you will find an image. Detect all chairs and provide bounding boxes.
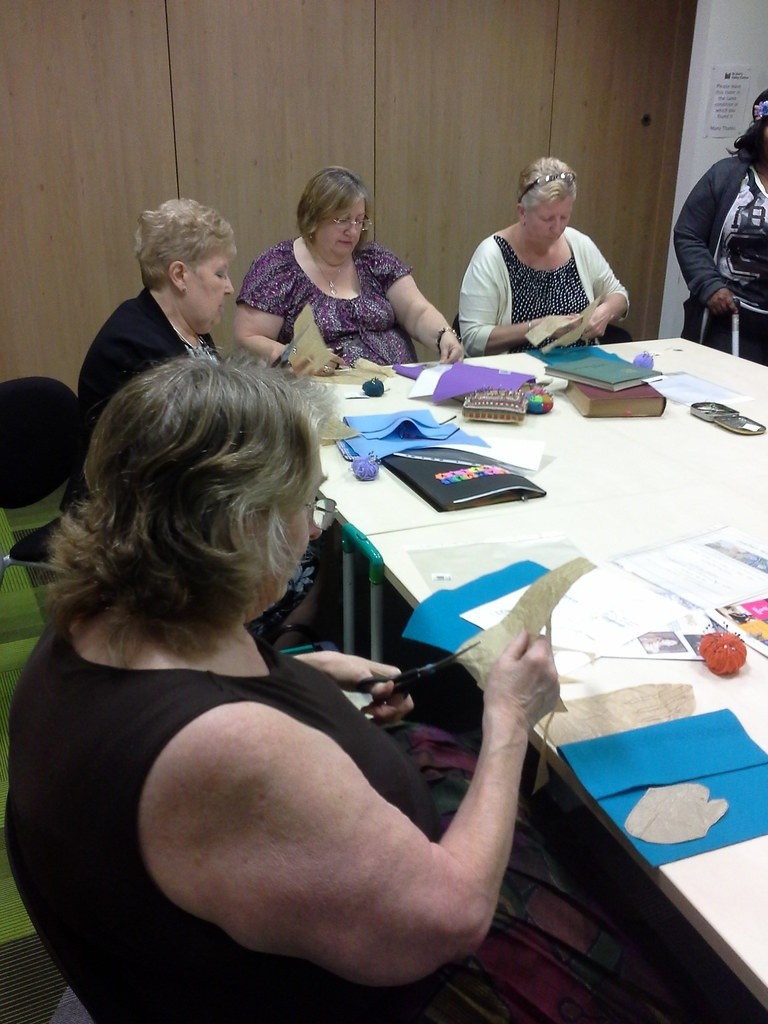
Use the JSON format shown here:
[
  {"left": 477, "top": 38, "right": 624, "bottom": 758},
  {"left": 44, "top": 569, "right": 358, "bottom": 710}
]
[{"left": 0, "top": 376, "right": 78, "bottom": 590}]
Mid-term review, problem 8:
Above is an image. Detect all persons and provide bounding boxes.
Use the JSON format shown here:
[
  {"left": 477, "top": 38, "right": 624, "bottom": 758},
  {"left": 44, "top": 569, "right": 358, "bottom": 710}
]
[
  {"left": 234, "top": 166, "right": 466, "bottom": 378},
  {"left": 458, "top": 154, "right": 633, "bottom": 355},
  {"left": 673, "top": 89, "right": 768, "bottom": 367},
  {"left": 5, "top": 359, "right": 704, "bottom": 1022},
  {"left": 57, "top": 197, "right": 321, "bottom": 644}
]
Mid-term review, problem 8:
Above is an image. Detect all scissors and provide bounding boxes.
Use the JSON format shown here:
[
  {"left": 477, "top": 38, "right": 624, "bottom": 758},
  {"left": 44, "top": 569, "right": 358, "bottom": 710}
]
[
  {"left": 268, "top": 321, "right": 313, "bottom": 372},
  {"left": 353, "top": 640, "right": 486, "bottom": 712}
]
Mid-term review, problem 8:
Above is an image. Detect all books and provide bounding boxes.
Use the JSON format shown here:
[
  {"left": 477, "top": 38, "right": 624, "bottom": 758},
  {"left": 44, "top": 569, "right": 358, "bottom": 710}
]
[{"left": 544, "top": 357, "right": 667, "bottom": 418}]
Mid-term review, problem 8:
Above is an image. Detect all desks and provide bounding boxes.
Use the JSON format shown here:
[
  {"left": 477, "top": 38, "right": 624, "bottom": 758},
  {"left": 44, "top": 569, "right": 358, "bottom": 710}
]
[{"left": 307, "top": 337, "right": 768, "bottom": 1010}]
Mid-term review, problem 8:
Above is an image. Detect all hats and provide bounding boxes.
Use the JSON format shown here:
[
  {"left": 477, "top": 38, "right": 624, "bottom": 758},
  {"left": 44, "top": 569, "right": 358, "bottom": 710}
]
[{"left": 752, "top": 89, "right": 768, "bottom": 122}]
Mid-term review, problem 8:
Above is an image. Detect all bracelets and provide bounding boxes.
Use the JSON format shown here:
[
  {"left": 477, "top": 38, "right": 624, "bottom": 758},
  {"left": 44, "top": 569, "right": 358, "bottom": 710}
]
[
  {"left": 437, "top": 328, "right": 461, "bottom": 355},
  {"left": 528, "top": 321, "right": 531, "bottom": 332},
  {"left": 287, "top": 347, "right": 297, "bottom": 366}
]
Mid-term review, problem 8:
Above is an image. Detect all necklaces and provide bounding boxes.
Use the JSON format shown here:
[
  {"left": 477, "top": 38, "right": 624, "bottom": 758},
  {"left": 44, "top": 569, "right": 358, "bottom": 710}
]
[{"left": 312, "top": 254, "right": 343, "bottom": 295}]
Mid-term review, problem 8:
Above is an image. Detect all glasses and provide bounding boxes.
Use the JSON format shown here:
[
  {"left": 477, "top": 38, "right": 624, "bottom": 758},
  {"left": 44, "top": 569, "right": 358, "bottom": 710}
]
[
  {"left": 305, "top": 498, "right": 338, "bottom": 531},
  {"left": 518, "top": 172, "right": 575, "bottom": 203},
  {"left": 330, "top": 215, "right": 372, "bottom": 232}
]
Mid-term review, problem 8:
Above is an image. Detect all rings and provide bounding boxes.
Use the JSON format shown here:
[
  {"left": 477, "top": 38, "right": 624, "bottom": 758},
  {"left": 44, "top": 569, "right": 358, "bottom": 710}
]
[{"left": 323, "top": 366, "right": 329, "bottom": 373}]
